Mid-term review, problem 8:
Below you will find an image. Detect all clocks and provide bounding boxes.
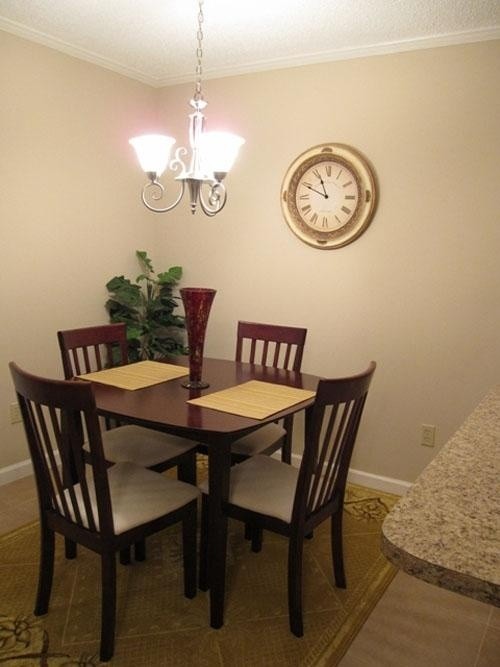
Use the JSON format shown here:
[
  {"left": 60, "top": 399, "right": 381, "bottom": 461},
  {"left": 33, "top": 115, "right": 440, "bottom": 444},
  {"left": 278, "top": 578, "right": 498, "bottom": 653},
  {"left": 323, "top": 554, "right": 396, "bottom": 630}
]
[{"left": 281, "top": 143, "right": 381, "bottom": 253}]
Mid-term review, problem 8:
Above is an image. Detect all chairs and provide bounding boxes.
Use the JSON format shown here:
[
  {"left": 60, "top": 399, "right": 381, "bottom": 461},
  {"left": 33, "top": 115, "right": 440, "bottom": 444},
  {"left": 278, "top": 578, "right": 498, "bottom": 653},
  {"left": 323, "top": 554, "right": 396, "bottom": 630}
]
[
  {"left": 53, "top": 320, "right": 199, "bottom": 565},
  {"left": 5, "top": 351, "right": 211, "bottom": 665},
  {"left": 190, "top": 355, "right": 385, "bottom": 639},
  {"left": 189, "top": 318, "right": 321, "bottom": 556}
]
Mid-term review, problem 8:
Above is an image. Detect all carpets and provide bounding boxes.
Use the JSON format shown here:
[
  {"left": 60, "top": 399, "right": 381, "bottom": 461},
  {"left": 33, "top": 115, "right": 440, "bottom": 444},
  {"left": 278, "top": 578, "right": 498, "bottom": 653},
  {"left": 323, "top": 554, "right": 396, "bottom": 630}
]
[{"left": 2, "top": 437, "right": 405, "bottom": 666}]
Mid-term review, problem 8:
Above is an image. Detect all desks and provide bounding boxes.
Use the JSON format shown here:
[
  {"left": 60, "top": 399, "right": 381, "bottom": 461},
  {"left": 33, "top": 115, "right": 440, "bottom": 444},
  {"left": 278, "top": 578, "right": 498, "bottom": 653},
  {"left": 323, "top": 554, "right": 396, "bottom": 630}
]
[{"left": 379, "top": 376, "right": 499, "bottom": 604}]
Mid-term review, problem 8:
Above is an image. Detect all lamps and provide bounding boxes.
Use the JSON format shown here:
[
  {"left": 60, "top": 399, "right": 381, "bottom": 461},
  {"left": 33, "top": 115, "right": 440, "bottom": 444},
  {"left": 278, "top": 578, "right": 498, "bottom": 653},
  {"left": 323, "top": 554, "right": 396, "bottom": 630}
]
[{"left": 122, "top": 0, "right": 246, "bottom": 217}]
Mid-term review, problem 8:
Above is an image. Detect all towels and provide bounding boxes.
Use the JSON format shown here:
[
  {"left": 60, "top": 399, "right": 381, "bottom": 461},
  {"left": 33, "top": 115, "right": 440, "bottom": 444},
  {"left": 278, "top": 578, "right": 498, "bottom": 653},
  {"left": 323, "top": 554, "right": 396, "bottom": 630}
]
[
  {"left": 63, "top": 354, "right": 189, "bottom": 392},
  {"left": 183, "top": 377, "right": 319, "bottom": 420}
]
